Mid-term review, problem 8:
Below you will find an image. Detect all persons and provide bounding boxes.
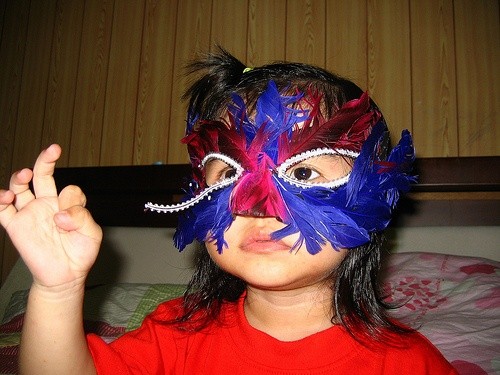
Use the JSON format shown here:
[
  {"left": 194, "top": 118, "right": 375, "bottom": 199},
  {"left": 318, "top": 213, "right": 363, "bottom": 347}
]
[{"left": 1, "top": 43, "right": 463, "bottom": 375}]
